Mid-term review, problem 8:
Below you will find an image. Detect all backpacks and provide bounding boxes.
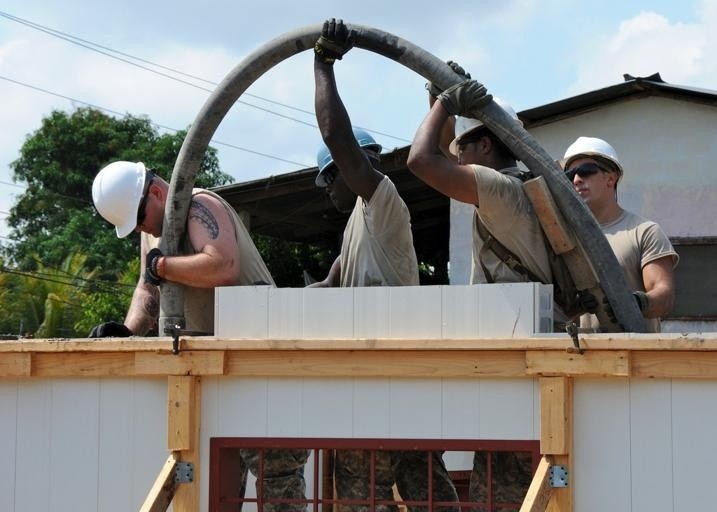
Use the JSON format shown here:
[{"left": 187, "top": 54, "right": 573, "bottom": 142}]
[{"left": 473, "top": 169, "right": 599, "bottom": 319}]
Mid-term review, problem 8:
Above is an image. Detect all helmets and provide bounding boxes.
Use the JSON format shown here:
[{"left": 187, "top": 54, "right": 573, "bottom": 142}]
[
  {"left": 90, "top": 161, "right": 147, "bottom": 239},
  {"left": 563, "top": 136, "right": 624, "bottom": 185},
  {"left": 447, "top": 97, "right": 524, "bottom": 156},
  {"left": 314, "top": 127, "right": 383, "bottom": 189}
]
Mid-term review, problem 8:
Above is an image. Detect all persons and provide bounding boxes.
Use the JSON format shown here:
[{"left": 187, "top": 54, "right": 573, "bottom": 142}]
[
  {"left": 408, "top": 58, "right": 568, "bottom": 511},
  {"left": 563, "top": 136, "right": 678, "bottom": 334},
  {"left": 307, "top": 19, "right": 462, "bottom": 511},
  {"left": 90, "top": 161, "right": 311, "bottom": 511}
]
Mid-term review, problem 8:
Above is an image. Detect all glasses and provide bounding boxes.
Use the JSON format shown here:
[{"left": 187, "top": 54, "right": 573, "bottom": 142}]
[
  {"left": 137, "top": 180, "right": 154, "bottom": 225},
  {"left": 456, "top": 136, "right": 487, "bottom": 155},
  {"left": 564, "top": 162, "right": 611, "bottom": 183},
  {"left": 319, "top": 168, "right": 347, "bottom": 186}
]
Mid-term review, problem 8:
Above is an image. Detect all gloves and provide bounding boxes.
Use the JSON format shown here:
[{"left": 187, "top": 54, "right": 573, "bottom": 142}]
[
  {"left": 313, "top": 17, "right": 357, "bottom": 65},
  {"left": 88, "top": 320, "right": 134, "bottom": 337},
  {"left": 142, "top": 248, "right": 166, "bottom": 286},
  {"left": 435, "top": 78, "right": 493, "bottom": 116},
  {"left": 427, "top": 60, "right": 471, "bottom": 101},
  {"left": 602, "top": 289, "right": 651, "bottom": 327}
]
